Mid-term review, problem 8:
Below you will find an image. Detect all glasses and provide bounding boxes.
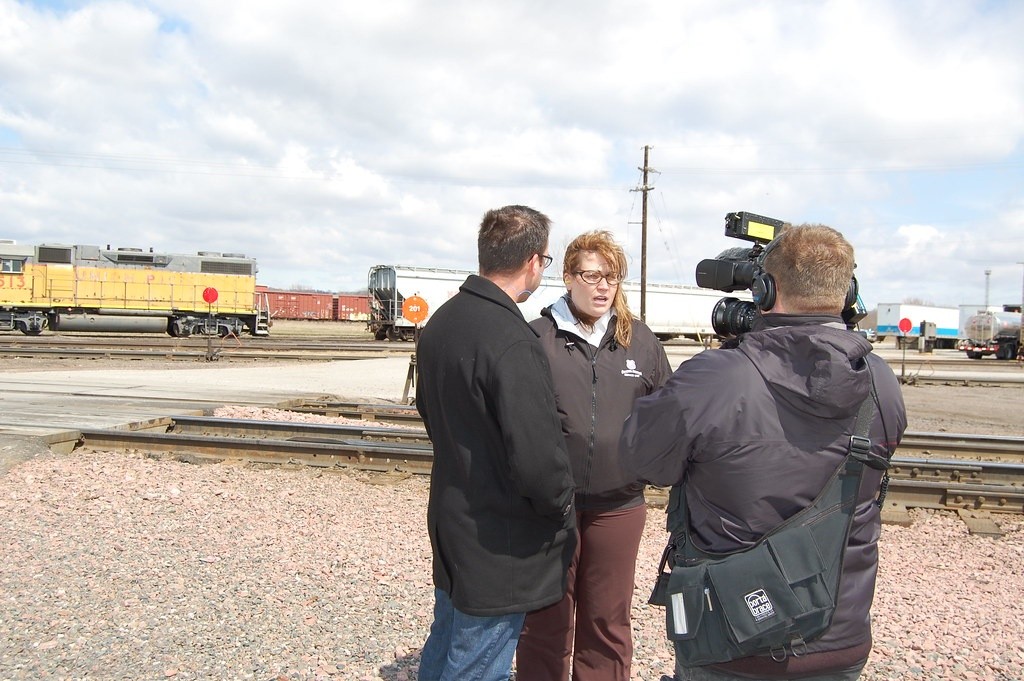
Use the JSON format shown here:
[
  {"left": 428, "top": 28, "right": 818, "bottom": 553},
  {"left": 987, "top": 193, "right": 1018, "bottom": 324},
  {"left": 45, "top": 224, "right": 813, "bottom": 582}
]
[
  {"left": 570, "top": 270, "right": 624, "bottom": 284},
  {"left": 528, "top": 253, "right": 553, "bottom": 268}
]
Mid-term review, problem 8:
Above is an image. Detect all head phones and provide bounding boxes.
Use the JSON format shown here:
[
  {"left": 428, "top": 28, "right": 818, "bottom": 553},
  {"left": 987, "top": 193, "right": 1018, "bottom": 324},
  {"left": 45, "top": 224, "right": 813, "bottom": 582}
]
[{"left": 751, "top": 233, "right": 859, "bottom": 311}]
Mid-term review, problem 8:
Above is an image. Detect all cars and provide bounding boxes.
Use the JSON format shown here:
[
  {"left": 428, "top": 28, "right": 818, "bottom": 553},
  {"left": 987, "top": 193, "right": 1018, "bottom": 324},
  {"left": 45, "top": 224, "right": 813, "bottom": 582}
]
[{"left": 859, "top": 328, "right": 877, "bottom": 343}]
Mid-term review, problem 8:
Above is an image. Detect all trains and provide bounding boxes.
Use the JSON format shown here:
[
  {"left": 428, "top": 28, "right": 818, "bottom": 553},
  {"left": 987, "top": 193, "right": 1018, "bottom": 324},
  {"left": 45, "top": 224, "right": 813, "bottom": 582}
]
[
  {"left": 0, "top": 238, "right": 271, "bottom": 337},
  {"left": 367, "top": 265, "right": 753, "bottom": 345}
]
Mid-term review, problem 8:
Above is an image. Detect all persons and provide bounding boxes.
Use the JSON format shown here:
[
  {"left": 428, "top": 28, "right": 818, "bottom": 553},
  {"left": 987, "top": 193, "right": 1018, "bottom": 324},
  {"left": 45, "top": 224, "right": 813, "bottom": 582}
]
[
  {"left": 622, "top": 225, "right": 909, "bottom": 681},
  {"left": 413, "top": 205, "right": 578, "bottom": 681},
  {"left": 515, "top": 232, "right": 674, "bottom": 681}
]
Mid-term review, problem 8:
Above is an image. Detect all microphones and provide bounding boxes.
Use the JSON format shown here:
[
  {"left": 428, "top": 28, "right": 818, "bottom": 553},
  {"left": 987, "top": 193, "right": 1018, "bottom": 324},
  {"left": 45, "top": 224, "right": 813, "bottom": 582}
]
[{"left": 712, "top": 247, "right": 766, "bottom": 263}]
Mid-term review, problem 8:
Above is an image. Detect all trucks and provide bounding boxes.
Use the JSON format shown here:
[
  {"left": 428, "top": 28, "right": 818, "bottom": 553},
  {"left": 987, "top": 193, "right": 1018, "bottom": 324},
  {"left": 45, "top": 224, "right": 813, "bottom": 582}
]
[{"left": 958, "top": 308, "right": 1024, "bottom": 360}]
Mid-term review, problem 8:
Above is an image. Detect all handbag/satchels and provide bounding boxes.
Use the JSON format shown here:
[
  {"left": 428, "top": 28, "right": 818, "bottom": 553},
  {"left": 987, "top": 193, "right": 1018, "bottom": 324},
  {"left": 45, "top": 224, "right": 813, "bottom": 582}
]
[{"left": 664, "top": 456, "right": 865, "bottom": 669}]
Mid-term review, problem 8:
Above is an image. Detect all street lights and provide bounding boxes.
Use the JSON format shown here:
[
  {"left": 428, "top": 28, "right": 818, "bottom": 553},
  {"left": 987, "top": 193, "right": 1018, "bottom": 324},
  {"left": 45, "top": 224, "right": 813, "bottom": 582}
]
[{"left": 1017, "top": 261, "right": 1024, "bottom": 313}]
